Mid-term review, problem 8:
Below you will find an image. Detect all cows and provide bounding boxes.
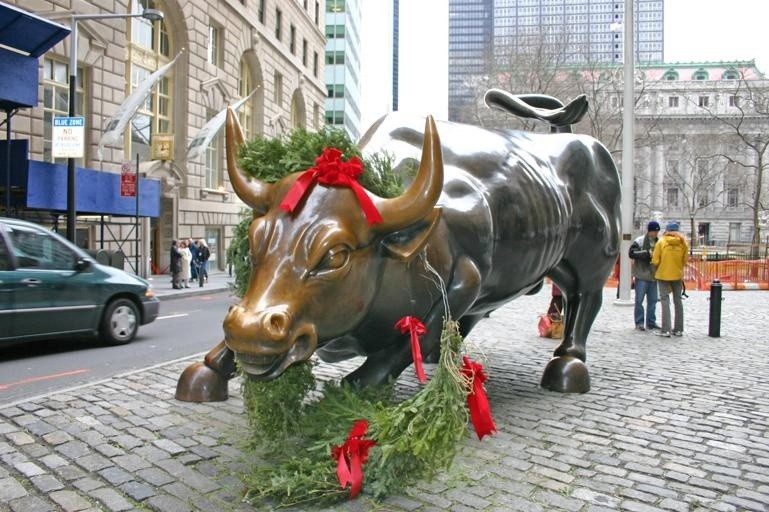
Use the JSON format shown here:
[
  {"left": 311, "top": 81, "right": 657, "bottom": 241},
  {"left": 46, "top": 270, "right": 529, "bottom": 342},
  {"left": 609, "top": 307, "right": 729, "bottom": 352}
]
[{"left": 174, "top": 87, "right": 624, "bottom": 415}]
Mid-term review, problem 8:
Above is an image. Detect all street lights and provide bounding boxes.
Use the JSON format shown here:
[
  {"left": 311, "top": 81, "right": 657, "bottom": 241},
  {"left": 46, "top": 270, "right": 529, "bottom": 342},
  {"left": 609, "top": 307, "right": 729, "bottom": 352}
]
[{"left": 67, "top": 9, "right": 163, "bottom": 244}]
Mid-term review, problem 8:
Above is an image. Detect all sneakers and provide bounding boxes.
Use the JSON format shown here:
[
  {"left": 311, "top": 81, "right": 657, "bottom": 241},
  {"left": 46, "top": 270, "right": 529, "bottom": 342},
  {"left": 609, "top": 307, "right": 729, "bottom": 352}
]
[
  {"left": 635, "top": 324, "right": 683, "bottom": 337},
  {"left": 171, "top": 277, "right": 209, "bottom": 289}
]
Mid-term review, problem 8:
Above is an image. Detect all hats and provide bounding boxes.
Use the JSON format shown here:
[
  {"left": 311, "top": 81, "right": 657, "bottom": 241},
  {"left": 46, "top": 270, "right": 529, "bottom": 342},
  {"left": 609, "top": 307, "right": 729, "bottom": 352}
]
[
  {"left": 647, "top": 221, "right": 660, "bottom": 231},
  {"left": 665, "top": 223, "right": 679, "bottom": 232}
]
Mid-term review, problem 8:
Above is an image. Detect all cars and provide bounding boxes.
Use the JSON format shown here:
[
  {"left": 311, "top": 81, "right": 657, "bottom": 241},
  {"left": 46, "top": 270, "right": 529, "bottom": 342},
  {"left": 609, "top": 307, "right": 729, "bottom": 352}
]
[{"left": 0, "top": 217, "right": 160, "bottom": 347}]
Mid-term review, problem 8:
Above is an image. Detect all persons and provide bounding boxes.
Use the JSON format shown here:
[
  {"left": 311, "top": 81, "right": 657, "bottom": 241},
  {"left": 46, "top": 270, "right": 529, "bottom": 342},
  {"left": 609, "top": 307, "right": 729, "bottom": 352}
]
[
  {"left": 170, "top": 238, "right": 211, "bottom": 289},
  {"left": 629, "top": 220, "right": 690, "bottom": 337},
  {"left": 548, "top": 282, "right": 566, "bottom": 314}
]
